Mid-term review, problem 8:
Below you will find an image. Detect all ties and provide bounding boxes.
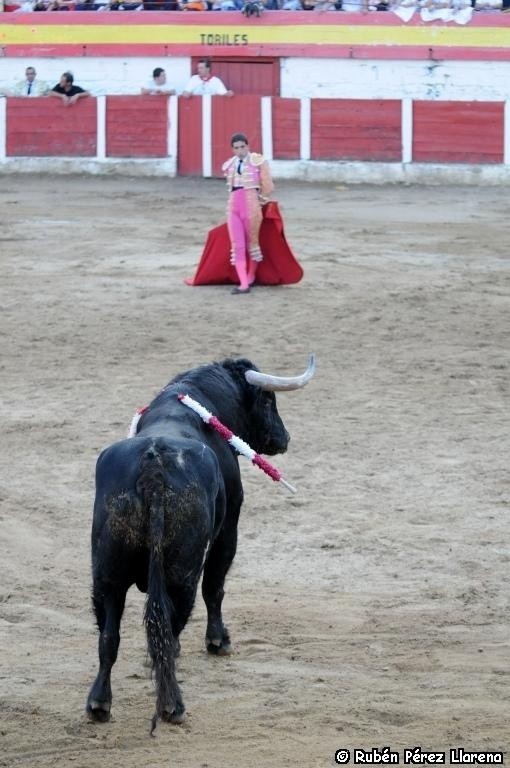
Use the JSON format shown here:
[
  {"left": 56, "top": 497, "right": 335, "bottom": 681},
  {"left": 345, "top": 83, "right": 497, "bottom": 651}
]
[{"left": 238, "top": 160, "right": 242, "bottom": 174}]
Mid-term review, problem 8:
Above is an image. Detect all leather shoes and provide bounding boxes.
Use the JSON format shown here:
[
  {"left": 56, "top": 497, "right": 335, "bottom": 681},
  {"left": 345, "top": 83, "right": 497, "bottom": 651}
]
[{"left": 232, "top": 280, "right": 255, "bottom": 293}]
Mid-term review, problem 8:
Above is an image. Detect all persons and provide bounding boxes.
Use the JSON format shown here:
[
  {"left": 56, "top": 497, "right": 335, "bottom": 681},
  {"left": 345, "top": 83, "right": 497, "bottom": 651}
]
[
  {"left": 47, "top": 72, "right": 90, "bottom": 106},
  {"left": 140, "top": 67, "right": 176, "bottom": 96},
  {"left": 220, "top": 133, "right": 273, "bottom": 294},
  {"left": 183, "top": 58, "right": 234, "bottom": 98},
  {"left": 10, "top": 67, "right": 48, "bottom": 96}
]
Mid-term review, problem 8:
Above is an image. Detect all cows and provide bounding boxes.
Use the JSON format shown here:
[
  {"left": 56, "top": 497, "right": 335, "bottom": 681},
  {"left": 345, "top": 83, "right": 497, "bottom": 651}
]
[{"left": 86, "top": 354, "right": 316, "bottom": 738}]
